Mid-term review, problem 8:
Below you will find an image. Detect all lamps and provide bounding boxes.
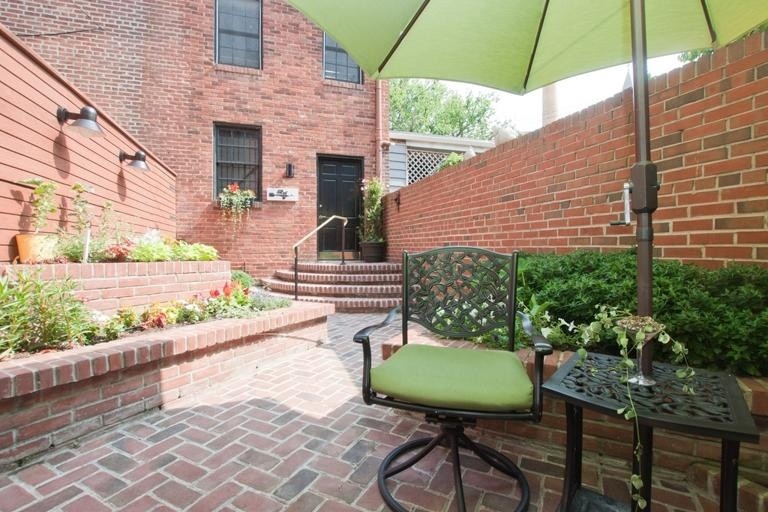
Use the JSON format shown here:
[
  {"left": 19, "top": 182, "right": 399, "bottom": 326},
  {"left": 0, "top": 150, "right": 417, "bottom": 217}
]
[
  {"left": 119, "top": 149, "right": 151, "bottom": 173},
  {"left": 56, "top": 105, "right": 107, "bottom": 140}
]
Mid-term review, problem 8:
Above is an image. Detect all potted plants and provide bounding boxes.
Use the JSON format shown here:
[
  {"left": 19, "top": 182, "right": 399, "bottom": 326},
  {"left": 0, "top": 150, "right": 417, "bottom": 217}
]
[
  {"left": 357, "top": 177, "right": 386, "bottom": 262},
  {"left": 241, "top": 190, "right": 256, "bottom": 208},
  {"left": 15, "top": 178, "right": 59, "bottom": 264}
]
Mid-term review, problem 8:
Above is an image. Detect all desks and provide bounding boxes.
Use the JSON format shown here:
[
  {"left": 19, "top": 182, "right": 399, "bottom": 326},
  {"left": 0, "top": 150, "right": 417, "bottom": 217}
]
[{"left": 541, "top": 348, "right": 761, "bottom": 512}]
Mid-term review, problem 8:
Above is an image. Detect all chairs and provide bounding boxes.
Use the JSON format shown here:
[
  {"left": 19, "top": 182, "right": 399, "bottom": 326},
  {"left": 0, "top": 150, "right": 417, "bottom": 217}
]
[{"left": 353, "top": 246, "right": 553, "bottom": 512}]
